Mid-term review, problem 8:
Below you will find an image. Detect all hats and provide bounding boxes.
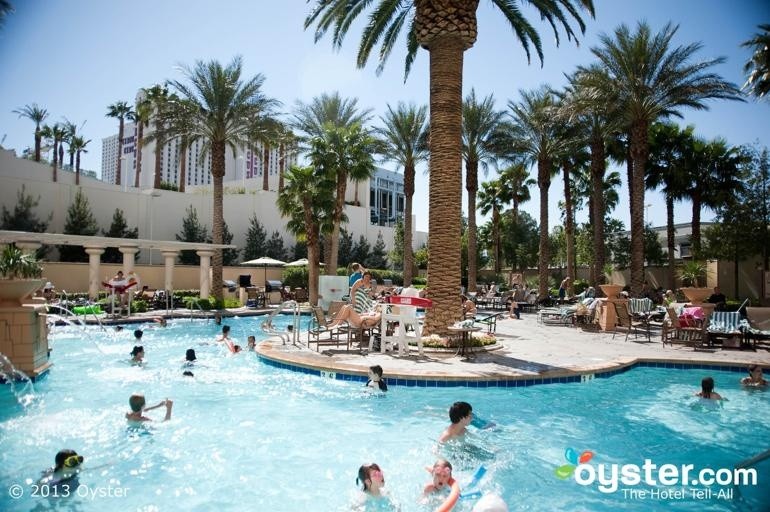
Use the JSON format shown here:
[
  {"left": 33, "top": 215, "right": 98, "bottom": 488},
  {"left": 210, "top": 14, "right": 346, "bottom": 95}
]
[{"left": 43, "top": 281, "right": 54, "bottom": 290}]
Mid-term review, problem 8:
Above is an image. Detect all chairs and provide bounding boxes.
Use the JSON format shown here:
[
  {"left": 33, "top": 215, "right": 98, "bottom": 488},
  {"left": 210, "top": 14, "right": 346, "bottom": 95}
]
[
  {"left": 246, "top": 287, "right": 266, "bottom": 310},
  {"left": 283, "top": 286, "right": 307, "bottom": 303},
  {"left": 474, "top": 312, "right": 503, "bottom": 335},
  {"left": 611, "top": 297, "right": 770, "bottom": 353},
  {"left": 307, "top": 300, "right": 393, "bottom": 354},
  {"left": 467, "top": 291, "right": 539, "bottom": 313},
  {"left": 537, "top": 298, "right": 599, "bottom": 327},
  {"left": 134, "top": 289, "right": 158, "bottom": 310}
]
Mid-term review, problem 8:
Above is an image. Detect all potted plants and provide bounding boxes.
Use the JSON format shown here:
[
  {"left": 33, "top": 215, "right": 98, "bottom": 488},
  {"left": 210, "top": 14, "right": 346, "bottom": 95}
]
[
  {"left": 0, "top": 242, "right": 47, "bottom": 306},
  {"left": 674, "top": 260, "right": 715, "bottom": 304},
  {"left": 599, "top": 262, "right": 626, "bottom": 299}
]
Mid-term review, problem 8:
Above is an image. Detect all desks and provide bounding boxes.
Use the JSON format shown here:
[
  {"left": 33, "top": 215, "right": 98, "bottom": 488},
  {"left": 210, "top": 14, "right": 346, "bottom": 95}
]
[{"left": 447, "top": 327, "right": 484, "bottom": 360}]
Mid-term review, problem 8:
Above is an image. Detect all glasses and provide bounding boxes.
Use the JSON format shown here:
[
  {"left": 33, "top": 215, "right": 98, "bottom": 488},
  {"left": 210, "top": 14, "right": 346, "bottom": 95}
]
[
  {"left": 371, "top": 471, "right": 383, "bottom": 478},
  {"left": 434, "top": 466, "right": 450, "bottom": 473},
  {"left": 64, "top": 456, "right": 83, "bottom": 467}
]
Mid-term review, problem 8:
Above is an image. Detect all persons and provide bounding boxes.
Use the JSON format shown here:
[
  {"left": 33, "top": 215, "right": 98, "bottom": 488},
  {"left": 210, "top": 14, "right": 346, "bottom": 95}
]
[
  {"left": 422, "top": 459, "right": 461, "bottom": 512},
  {"left": 708, "top": 286, "right": 726, "bottom": 311},
  {"left": 160, "top": 314, "right": 294, "bottom": 356},
  {"left": 459, "top": 266, "right": 678, "bottom": 324},
  {"left": 695, "top": 377, "right": 724, "bottom": 401},
  {"left": 107, "top": 268, "right": 164, "bottom": 310},
  {"left": 125, "top": 393, "right": 173, "bottom": 424},
  {"left": 356, "top": 462, "right": 393, "bottom": 500},
  {"left": 323, "top": 262, "right": 427, "bottom": 333},
  {"left": 35, "top": 449, "right": 85, "bottom": 495},
  {"left": 366, "top": 364, "right": 387, "bottom": 392},
  {"left": 438, "top": 401, "right": 473, "bottom": 444},
  {"left": 42, "top": 282, "right": 56, "bottom": 304},
  {"left": 133, "top": 329, "right": 143, "bottom": 342},
  {"left": 184, "top": 348, "right": 197, "bottom": 362},
  {"left": 129, "top": 345, "right": 145, "bottom": 362},
  {"left": 739, "top": 364, "right": 769, "bottom": 387}
]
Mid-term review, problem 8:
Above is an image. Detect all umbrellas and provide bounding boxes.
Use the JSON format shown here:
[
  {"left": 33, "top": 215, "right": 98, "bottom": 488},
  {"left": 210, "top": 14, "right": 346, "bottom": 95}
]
[
  {"left": 284, "top": 257, "right": 327, "bottom": 288},
  {"left": 239, "top": 255, "right": 286, "bottom": 291}
]
